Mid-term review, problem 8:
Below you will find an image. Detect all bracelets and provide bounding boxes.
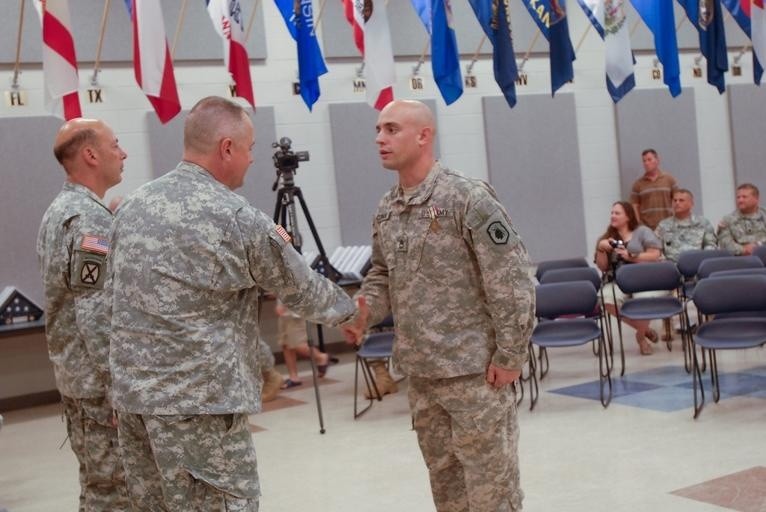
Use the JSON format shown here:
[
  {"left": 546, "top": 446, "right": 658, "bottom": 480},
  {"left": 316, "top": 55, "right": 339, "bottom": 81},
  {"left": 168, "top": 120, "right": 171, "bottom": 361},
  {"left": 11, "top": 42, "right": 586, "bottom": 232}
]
[
  {"left": 629, "top": 251, "right": 633, "bottom": 261},
  {"left": 596, "top": 247, "right": 606, "bottom": 253}
]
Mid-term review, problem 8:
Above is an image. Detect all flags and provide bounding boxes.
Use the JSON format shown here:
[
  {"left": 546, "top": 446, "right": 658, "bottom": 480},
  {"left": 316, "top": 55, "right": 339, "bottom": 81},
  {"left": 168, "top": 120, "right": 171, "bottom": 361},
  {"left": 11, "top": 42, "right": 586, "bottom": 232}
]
[
  {"left": 201, "top": 1, "right": 256, "bottom": 114},
  {"left": 343, "top": 1, "right": 396, "bottom": 110},
  {"left": 578, "top": 1, "right": 638, "bottom": 105},
  {"left": 630, "top": 1, "right": 684, "bottom": 100},
  {"left": 407, "top": 0, "right": 463, "bottom": 108},
  {"left": 719, "top": 0, "right": 766, "bottom": 91},
  {"left": 468, "top": 1, "right": 521, "bottom": 111},
  {"left": 273, "top": 0, "right": 328, "bottom": 112},
  {"left": 521, "top": 1, "right": 579, "bottom": 97},
  {"left": 122, "top": 0, "right": 182, "bottom": 127},
  {"left": 675, "top": 1, "right": 729, "bottom": 95},
  {"left": 31, "top": 0, "right": 81, "bottom": 124}
]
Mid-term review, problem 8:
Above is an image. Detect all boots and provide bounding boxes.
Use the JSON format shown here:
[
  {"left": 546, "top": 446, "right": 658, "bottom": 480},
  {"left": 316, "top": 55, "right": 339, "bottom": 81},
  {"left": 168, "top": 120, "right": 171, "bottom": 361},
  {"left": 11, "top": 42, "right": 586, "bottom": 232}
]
[
  {"left": 364, "top": 360, "right": 398, "bottom": 398},
  {"left": 262, "top": 369, "right": 282, "bottom": 402}
]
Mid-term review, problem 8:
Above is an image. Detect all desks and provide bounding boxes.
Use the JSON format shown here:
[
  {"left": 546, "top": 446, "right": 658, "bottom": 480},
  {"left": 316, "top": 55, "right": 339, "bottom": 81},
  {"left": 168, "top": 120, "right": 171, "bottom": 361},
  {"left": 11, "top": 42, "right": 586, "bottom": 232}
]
[{"left": 0, "top": 278, "right": 364, "bottom": 363}]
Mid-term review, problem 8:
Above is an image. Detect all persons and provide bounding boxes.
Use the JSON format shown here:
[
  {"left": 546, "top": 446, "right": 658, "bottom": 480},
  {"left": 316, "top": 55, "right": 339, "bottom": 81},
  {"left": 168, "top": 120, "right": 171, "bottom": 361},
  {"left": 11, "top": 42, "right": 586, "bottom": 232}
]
[
  {"left": 104, "top": 97, "right": 371, "bottom": 512},
  {"left": 629, "top": 148, "right": 677, "bottom": 230},
  {"left": 359, "top": 254, "right": 398, "bottom": 401},
  {"left": 35, "top": 117, "right": 139, "bottom": 511},
  {"left": 653, "top": 188, "right": 718, "bottom": 335},
  {"left": 341, "top": 98, "right": 539, "bottom": 512},
  {"left": 594, "top": 202, "right": 665, "bottom": 359},
  {"left": 716, "top": 183, "right": 765, "bottom": 258},
  {"left": 252, "top": 328, "right": 282, "bottom": 401},
  {"left": 273, "top": 288, "right": 332, "bottom": 389}
]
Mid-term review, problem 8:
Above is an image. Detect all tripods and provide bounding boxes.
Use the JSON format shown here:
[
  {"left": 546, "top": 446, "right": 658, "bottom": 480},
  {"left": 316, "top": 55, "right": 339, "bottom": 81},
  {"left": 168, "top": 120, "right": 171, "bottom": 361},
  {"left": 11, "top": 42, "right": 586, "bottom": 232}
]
[{"left": 274, "top": 186, "right": 382, "bottom": 434}]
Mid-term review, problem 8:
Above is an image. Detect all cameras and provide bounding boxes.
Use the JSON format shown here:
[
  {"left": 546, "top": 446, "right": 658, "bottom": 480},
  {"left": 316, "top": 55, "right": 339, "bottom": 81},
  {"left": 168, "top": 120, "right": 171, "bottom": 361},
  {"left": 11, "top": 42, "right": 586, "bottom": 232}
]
[
  {"left": 611, "top": 240, "right": 623, "bottom": 248},
  {"left": 272, "top": 136, "right": 309, "bottom": 170}
]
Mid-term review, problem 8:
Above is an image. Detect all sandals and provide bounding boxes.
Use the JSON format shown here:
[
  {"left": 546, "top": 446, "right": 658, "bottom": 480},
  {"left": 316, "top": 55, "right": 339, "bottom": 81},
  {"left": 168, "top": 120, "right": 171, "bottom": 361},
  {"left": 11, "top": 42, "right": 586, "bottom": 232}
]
[{"left": 635, "top": 329, "right": 659, "bottom": 355}]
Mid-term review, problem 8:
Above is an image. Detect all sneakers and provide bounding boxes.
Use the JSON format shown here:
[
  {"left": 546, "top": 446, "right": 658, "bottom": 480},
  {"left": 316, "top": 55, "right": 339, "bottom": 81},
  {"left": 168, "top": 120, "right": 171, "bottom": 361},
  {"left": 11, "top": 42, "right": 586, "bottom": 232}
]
[{"left": 678, "top": 325, "right": 696, "bottom": 335}]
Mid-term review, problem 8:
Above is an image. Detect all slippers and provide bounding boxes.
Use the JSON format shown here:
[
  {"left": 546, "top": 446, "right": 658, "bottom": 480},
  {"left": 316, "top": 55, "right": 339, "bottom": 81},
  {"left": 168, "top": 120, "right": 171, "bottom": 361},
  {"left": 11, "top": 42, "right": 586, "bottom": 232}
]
[
  {"left": 317, "top": 352, "right": 330, "bottom": 377},
  {"left": 281, "top": 378, "right": 302, "bottom": 389}
]
